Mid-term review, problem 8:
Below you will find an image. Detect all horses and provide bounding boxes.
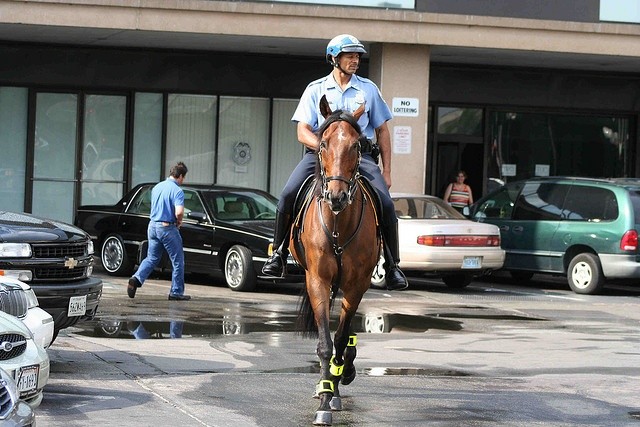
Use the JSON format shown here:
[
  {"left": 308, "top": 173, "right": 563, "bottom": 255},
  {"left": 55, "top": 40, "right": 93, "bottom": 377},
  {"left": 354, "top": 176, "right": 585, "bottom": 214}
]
[{"left": 288, "top": 94, "right": 380, "bottom": 425}]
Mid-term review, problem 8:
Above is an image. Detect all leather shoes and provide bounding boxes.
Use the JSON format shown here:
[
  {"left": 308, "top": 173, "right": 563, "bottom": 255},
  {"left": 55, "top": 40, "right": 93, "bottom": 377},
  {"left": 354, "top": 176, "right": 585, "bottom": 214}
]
[
  {"left": 169, "top": 294, "right": 190, "bottom": 301},
  {"left": 127, "top": 277, "right": 137, "bottom": 299}
]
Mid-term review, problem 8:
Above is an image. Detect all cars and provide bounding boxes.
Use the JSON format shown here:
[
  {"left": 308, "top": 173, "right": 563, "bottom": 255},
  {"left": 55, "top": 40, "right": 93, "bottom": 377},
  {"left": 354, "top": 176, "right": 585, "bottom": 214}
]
[
  {"left": 371, "top": 192, "right": 507, "bottom": 290},
  {"left": 74, "top": 180, "right": 307, "bottom": 293},
  {"left": 0, "top": 311, "right": 50, "bottom": 410},
  {"left": 0, "top": 368, "right": 36, "bottom": 427},
  {"left": 0, "top": 278, "right": 55, "bottom": 348}
]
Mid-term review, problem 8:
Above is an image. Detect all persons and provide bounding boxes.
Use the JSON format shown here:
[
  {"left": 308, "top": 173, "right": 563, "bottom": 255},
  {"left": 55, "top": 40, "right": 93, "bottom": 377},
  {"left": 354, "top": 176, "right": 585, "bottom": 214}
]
[
  {"left": 262, "top": 35, "right": 407, "bottom": 289},
  {"left": 127, "top": 162, "right": 190, "bottom": 301},
  {"left": 444, "top": 171, "right": 473, "bottom": 214}
]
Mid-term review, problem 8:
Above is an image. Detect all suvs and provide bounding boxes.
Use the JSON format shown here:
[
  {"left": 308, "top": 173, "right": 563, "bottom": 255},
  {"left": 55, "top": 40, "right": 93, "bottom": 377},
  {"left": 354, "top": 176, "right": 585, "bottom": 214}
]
[
  {"left": 0, "top": 210, "right": 104, "bottom": 349},
  {"left": 459, "top": 176, "right": 640, "bottom": 295}
]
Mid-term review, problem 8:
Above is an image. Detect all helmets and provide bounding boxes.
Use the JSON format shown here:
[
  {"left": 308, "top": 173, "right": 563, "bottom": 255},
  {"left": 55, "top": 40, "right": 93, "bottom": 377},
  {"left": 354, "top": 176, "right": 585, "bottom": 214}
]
[{"left": 326, "top": 34, "right": 368, "bottom": 75}]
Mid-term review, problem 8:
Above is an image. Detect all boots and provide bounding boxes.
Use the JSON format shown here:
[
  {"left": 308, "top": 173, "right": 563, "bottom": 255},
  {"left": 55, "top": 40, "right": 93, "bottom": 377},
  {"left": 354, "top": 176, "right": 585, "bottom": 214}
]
[
  {"left": 383, "top": 220, "right": 408, "bottom": 291},
  {"left": 262, "top": 208, "right": 290, "bottom": 278}
]
